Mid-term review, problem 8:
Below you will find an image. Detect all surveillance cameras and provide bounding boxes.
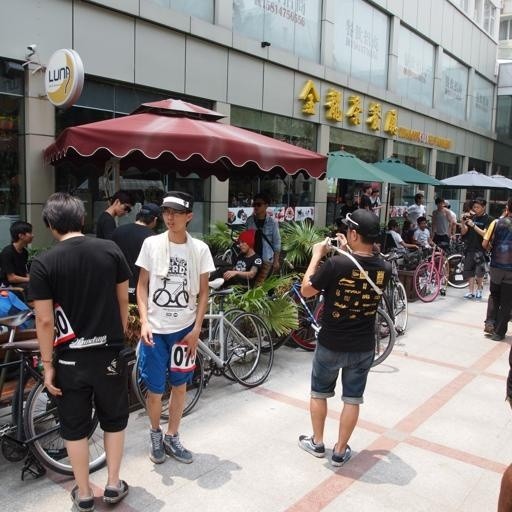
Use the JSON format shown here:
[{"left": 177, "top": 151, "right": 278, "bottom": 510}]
[{"left": 26, "top": 43, "right": 37, "bottom": 52}]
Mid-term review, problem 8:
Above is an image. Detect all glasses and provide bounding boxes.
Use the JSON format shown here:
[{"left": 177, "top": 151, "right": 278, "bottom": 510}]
[
  {"left": 124, "top": 204, "right": 132, "bottom": 213},
  {"left": 252, "top": 202, "right": 264, "bottom": 207}
]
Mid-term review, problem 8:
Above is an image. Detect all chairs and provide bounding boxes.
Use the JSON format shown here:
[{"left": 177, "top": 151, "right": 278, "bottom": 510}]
[
  {"left": 0, "top": 277, "right": 34, "bottom": 309},
  {"left": 408, "top": 228, "right": 429, "bottom": 256},
  {"left": 387, "top": 233, "right": 418, "bottom": 257},
  {"left": 226, "top": 260, "right": 272, "bottom": 291}
]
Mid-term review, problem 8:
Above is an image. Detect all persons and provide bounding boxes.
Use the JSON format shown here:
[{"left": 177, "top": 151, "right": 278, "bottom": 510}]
[
  {"left": 135, "top": 189, "right": 217, "bottom": 464},
  {"left": 208, "top": 229, "right": 263, "bottom": 291},
  {"left": 296, "top": 209, "right": 392, "bottom": 467},
  {"left": 0, "top": 221, "right": 34, "bottom": 299},
  {"left": 112, "top": 204, "right": 162, "bottom": 305},
  {"left": 497, "top": 346, "right": 512, "bottom": 512},
  {"left": 96, "top": 189, "right": 135, "bottom": 243},
  {"left": 334, "top": 185, "right": 512, "bottom": 341},
  {"left": 29, "top": 192, "right": 134, "bottom": 512},
  {"left": 245, "top": 194, "right": 282, "bottom": 287}
]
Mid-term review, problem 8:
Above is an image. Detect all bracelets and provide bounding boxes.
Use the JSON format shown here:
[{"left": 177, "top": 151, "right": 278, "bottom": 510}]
[{"left": 39, "top": 359, "right": 53, "bottom": 364}]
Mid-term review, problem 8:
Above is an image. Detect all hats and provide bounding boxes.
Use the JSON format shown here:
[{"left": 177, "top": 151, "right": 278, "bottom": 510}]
[
  {"left": 142, "top": 203, "right": 164, "bottom": 224},
  {"left": 347, "top": 208, "right": 381, "bottom": 239},
  {"left": 239, "top": 228, "right": 255, "bottom": 248},
  {"left": 159, "top": 190, "right": 195, "bottom": 213}
]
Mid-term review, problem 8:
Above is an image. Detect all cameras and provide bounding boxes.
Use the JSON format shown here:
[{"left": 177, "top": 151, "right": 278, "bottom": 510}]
[{"left": 329, "top": 239, "right": 339, "bottom": 248}]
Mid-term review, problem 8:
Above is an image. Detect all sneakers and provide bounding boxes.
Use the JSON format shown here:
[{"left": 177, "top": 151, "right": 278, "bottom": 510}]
[
  {"left": 492, "top": 331, "right": 506, "bottom": 341},
  {"left": 331, "top": 441, "right": 352, "bottom": 467},
  {"left": 484, "top": 322, "right": 496, "bottom": 336},
  {"left": 148, "top": 426, "right": 166, "bottom": 464},
  {"left": 464, "top": 293, "right": 476, "bottom": 299},
  {"left": 298, "top": 434, "right": 325, "bottom": 458},
  {"left": 163, "top": 430, "right": 194, "bottom": 465},
  {"left": 70, "top": 484, "right": 95, "bottom": 511},
  {"left": 103, "top": 477, "right": 130, "bottom": 505},
  {"left": 476, "top": 290, "right": 483, "bottom": 300}
]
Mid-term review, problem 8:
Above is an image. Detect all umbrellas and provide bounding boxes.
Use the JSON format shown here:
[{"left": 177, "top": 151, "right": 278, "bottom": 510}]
[
  {"left": 367, "top": 155, "right": 447, "bottom": 254},
  {"left": 488, "top": 174, "right": 511, "bottom": 192},
  {"left": 327, "top": 147, "right": 407, "bottom": 233},
  {"left": 440, "top": 168, "right": 507, "bottom": 203}
]
